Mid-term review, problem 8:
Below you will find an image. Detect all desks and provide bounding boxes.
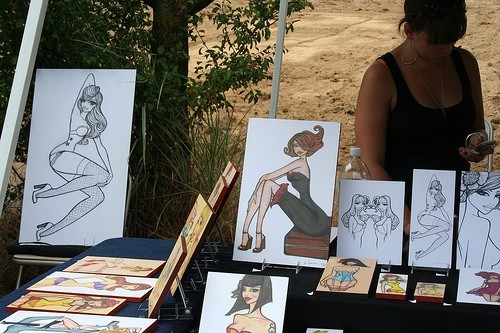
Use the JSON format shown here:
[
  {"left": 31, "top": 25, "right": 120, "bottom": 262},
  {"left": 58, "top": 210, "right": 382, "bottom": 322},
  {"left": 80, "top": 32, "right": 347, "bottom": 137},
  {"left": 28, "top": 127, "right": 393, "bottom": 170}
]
[{"left": 0, "top": 237, "right": 500, "bottom": 333}]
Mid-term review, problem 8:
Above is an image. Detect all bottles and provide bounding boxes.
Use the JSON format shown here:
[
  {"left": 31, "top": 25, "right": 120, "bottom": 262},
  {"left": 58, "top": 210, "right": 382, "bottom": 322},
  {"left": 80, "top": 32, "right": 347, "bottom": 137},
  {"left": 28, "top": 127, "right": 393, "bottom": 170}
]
[{"left": 339, "top": 147, "right": 371, "bottom": 180}]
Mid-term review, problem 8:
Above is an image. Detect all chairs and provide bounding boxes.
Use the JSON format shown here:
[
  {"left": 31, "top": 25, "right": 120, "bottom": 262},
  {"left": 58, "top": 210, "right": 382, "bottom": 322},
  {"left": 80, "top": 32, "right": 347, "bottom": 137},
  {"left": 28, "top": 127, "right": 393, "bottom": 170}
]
[{"left": 12, "top": 173, "right": 133, "bottom": 290}]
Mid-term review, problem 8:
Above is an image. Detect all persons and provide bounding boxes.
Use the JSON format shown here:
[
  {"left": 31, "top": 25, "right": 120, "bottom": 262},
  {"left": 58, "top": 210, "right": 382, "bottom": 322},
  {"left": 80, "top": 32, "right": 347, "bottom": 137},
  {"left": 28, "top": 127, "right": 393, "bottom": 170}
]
[{"left": 355, "top": 0, "right": 489, "bottom": 266}]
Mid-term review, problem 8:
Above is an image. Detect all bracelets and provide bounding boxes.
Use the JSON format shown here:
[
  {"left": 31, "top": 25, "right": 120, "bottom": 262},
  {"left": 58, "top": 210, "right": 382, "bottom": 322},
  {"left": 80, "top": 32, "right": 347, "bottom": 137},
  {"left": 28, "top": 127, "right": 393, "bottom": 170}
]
[{"left": 465, "top": 132, "right": 486, "bottom": 148}]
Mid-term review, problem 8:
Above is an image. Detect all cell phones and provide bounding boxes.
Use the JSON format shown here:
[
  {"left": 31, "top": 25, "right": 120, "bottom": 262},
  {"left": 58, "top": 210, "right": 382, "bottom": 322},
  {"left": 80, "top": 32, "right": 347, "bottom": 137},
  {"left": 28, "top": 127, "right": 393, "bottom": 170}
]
[{"left": 467, "top": 140, "right": 496, "bottom": 155}]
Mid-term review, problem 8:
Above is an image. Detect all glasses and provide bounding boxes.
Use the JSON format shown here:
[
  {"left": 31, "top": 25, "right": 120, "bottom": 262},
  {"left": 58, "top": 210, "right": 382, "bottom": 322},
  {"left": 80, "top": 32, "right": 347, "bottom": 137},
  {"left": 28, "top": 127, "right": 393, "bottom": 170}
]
[{"left": 431, "top": 37, "right": 456, "bottom": 44}]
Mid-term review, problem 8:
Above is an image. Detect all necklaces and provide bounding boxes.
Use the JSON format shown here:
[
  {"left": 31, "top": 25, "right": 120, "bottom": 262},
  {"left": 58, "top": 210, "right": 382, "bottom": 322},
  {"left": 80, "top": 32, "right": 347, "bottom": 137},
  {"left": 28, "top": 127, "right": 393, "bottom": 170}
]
[{"left": 405, "top": 46, "right": 447, "bottom": 120}]
